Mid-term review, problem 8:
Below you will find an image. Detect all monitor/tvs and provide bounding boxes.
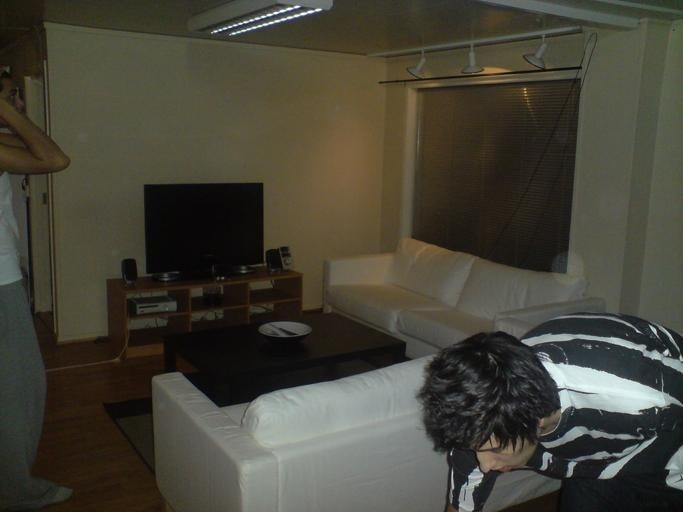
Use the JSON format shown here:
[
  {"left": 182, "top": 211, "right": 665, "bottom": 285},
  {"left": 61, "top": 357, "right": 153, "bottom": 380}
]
[{"left": 144, "top": 183, "right": 264, "bottom": 282}]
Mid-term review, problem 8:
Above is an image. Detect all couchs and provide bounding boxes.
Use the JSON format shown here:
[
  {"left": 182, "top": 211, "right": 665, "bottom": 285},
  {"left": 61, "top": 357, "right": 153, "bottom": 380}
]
[
  {"left": 321, "top": 251, "right": 607, "bottom": 357},
  {"left": 151, "top": 315, "right": 564, "bottom": 512}
]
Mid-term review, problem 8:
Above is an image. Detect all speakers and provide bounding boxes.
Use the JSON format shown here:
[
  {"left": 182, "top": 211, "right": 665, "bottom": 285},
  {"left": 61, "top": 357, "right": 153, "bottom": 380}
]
[
  {"left": 122, "top": 259, "right": 137, "bottom": 289},
  {"left": 267, "top": 250, "right": 283, "bottom": 275}
]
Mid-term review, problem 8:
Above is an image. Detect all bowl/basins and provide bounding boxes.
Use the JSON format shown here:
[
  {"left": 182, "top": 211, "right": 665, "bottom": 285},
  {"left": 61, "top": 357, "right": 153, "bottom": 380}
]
[{"left": 258, "top": 321, "right": 312, "bottom": 344}]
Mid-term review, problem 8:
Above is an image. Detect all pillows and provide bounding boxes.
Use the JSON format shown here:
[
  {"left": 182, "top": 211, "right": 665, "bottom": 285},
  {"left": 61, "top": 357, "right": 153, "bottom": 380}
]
[
  {"left": 388, "top": 237, "right": 477, "bottom": 310},
  {"left": 239, "top": 351, "right": 441, "bottom": 452},
  {"left": 455, "top": 257, "right": 590, "bottom": 323}
]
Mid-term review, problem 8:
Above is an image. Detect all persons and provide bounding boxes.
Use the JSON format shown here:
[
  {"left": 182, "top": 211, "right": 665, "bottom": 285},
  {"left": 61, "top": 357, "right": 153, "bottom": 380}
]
[
  {"left": 0, "top": 66, "right": 71, "bottom": 509},
  {"left": 415, "top": 311, "right": 683, "bottom": 511}
]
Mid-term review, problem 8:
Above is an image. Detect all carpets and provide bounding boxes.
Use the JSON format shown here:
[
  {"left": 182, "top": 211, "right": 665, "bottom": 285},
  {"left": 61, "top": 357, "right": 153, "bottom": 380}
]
[{"left": 101, "top": 396, "right": 154, "bottom": 474}]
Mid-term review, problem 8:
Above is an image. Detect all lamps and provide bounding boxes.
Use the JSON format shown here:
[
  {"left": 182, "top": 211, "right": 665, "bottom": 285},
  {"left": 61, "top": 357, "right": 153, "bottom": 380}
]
[
  {"left": 367, "top": 25, "right": 582, "bottom": 79},
  {"left": 186, "top": 0, "right": 334, "bottom": 38}
]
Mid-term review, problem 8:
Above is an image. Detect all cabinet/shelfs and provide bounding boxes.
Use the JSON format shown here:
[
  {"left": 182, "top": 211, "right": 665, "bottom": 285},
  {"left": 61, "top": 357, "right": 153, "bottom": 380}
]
[{"left": 107, "top": 264, "right": 304, "bottom": 362}]
